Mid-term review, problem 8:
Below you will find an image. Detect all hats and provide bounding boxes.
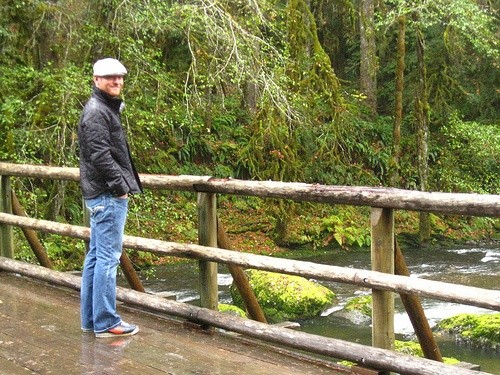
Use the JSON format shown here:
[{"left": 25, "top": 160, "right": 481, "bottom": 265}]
[{"left": 93, "top": 58, "right": 127, "bottom": 77}]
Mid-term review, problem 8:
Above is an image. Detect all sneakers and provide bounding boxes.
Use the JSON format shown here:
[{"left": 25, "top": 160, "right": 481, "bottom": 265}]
[
  {"left": 95, "top": 320, "right": 139, "bottom": 337},
  {"left": 83, "top": 328, "right": 94, "bottom": 332}
]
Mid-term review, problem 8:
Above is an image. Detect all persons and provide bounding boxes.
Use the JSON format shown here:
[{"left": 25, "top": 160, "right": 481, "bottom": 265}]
[{"left": 77, "top": 58, "right": 143, "bottom": 338}]
